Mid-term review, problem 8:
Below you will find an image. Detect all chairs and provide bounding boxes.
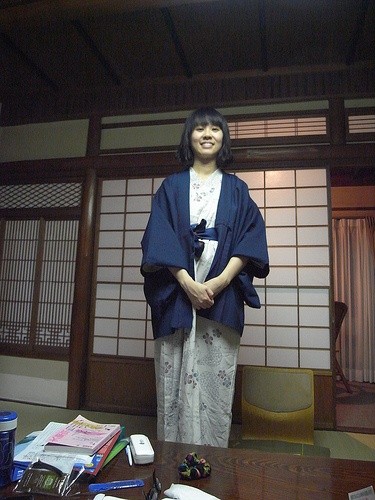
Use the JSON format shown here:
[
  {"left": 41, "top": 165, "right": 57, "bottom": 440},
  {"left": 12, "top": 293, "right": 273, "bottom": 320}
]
[
  {"left": 242, "top": 366, "right": 314, "bottom": 456},
  {"left": 335, "top": 301, "right": 352, "bottom": 395}
]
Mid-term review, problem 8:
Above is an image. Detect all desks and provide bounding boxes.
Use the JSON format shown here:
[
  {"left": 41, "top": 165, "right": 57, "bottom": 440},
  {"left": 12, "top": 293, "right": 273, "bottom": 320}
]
[{"left": 0, "top": 440, "right": 375, "bottom": 500}]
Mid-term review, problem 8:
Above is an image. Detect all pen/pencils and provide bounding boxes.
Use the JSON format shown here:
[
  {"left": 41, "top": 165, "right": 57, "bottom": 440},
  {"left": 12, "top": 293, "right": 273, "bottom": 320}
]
[{"left": 126, "top": 445, "right": 133, "bottom": 466}]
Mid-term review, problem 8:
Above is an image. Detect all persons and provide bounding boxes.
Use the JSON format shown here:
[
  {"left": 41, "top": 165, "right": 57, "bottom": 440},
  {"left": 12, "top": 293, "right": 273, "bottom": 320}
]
[{"left": 139, "top": 105, "right": 270, "bottom": 447}]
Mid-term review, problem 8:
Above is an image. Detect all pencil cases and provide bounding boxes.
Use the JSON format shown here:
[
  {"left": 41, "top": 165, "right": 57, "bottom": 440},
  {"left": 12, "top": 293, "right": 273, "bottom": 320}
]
[{"left": 130, "top": 433, "right": 154, "bottom": 465}]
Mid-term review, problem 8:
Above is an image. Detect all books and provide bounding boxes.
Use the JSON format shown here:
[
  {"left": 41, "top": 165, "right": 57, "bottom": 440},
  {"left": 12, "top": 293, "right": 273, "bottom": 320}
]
[{"left": 14, "top": 415, "right": 129, "bottom": 477}]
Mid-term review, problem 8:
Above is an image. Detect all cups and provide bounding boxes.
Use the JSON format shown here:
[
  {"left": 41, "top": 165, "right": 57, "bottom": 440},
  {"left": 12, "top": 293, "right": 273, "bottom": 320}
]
[{"left": 0, "top": 410, "right": 18, "bottom": 488}]
